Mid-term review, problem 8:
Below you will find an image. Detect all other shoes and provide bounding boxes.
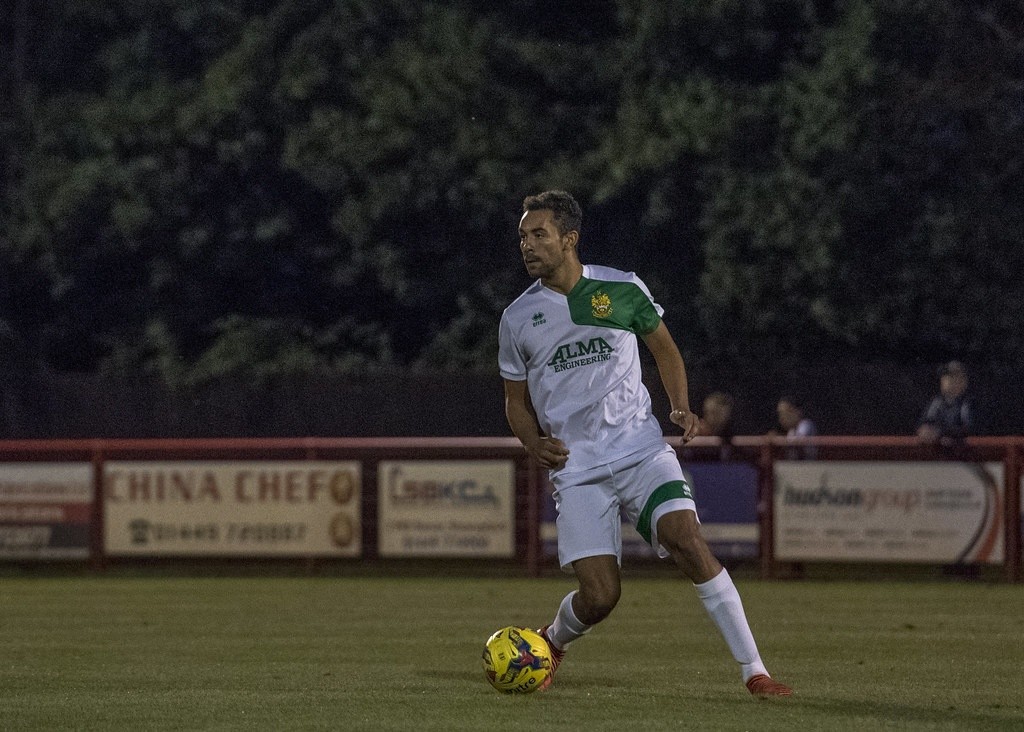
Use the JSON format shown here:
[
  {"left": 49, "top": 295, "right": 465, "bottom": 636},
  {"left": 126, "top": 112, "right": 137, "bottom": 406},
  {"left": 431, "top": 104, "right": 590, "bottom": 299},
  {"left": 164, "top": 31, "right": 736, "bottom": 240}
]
[
  {"left": 535, "top": 624, "right": 567, "bottom": 693},
  {"left": 747, "top": 674, "right": 795, "bottom": 700}
]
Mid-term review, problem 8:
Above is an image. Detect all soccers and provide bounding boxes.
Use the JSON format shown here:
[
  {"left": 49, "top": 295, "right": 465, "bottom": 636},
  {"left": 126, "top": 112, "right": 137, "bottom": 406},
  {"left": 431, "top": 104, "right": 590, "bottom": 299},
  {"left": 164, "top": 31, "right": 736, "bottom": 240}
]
[{"left": 483, "top": 625, "right": 553, "bottom": 695}]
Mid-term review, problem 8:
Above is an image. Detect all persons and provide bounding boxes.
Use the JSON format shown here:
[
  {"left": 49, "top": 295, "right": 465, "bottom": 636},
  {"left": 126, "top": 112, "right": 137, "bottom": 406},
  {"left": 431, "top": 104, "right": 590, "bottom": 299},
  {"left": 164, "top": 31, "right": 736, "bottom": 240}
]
[
  {"left": 498, "top": 190, "right": 793, "bottom": 696},
  {"left": 915, "top": 361, "right": 976, "bottom": 444},
  {"left": 775, "top": 393, "right": 818, "bottom": 460},
  {"left": 696, "top": 391, "right": 736, "bottom": 462}
]
